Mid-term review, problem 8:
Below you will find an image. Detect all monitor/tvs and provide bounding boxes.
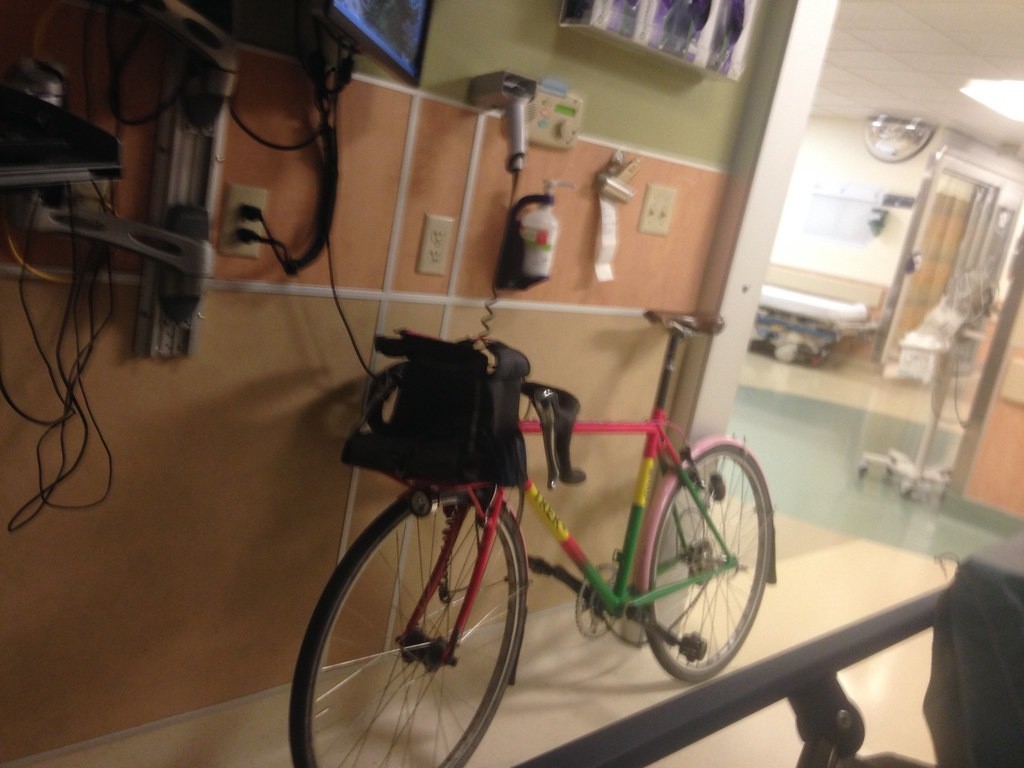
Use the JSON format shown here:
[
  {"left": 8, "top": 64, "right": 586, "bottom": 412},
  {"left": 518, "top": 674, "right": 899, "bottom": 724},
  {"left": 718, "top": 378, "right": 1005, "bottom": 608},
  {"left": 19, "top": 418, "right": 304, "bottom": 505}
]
[{"left": 328, "top": 0, "right": 433, "bottom": 86}]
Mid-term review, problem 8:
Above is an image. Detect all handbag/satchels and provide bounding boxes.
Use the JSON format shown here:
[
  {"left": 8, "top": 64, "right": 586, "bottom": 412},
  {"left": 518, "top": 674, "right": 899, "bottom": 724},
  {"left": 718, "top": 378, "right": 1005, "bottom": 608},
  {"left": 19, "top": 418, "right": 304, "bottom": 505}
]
[{"left": 339, "top": 337, "right": 531, "bottom": 488}]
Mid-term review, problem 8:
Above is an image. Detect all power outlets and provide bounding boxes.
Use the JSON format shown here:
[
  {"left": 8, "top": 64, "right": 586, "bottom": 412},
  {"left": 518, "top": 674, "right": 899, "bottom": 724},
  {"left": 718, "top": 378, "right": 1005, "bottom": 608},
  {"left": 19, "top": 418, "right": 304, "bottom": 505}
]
[
  {"left": 218, "top": 184, "right": 269, "bottom": 259},
  {"left": 638, "top": 185, "right": 675, "bottom": 236},
  {"left": 416, "top": 214, "right": 454, "bottom": 276}
]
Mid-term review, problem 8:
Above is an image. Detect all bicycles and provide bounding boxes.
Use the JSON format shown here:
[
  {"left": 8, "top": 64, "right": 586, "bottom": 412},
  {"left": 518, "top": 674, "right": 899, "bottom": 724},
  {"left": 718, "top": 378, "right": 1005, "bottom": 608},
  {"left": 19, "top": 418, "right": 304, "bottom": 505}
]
[{"left": 287, "top": 309, "right": 776, "bottom": 767}]
